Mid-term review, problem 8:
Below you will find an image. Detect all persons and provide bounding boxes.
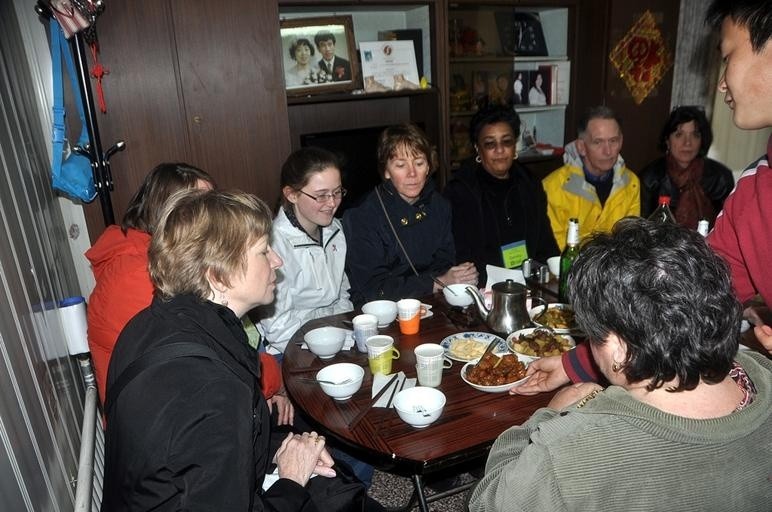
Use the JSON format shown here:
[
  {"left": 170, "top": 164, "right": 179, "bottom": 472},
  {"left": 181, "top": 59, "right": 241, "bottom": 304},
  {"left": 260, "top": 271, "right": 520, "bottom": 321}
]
[
  {"left": 99, "top": 187, "right": 385, "bottom": 512},
  {"left": 468, "top": 212, "right": 772, "bottom": 512},
  {"left": 678, "top": 0, "right": 772, "bottom": 363},
  {"left": 83, "top": 160, "right": 295, "bottom": 430},
  {"left": 316, "top": 29, "right": 352, "bottom": 84},
  {"left": 341, "top": 117, "right": 480, "bottom": 308},
  {"left": 637, "top": 103, "right": 736, "bottom": 243},
  {"left": 253, "top": 143, "right": 378, "bottom": 494},
  {"left": 540, "top": 103, "right": 645, "bottom": 254},
  {"left": 285, "top": 37, "right": 319, "bottom": 86},
  {"left": 444, "top": 101, "right": 567, "bottom": 290},
  {"left": 528, "top": 72, "right": 547, "bottom": 107},
  {"left": 513, "top": 71, "right": 527, "bottom": 105}
]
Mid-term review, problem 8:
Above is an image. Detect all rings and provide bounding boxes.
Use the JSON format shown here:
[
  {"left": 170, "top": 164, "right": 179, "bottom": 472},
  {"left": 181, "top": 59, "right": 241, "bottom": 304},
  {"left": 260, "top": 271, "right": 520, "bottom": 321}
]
[{"left": 307, "top": 435, "right": 318, "bottom": 441}]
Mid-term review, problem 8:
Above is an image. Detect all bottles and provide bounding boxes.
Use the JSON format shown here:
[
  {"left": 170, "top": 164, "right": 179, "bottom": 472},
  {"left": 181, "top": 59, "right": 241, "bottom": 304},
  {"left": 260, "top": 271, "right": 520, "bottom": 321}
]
[
  {"left": 522, "top": 257, "right": 550, "bottom": 284},
  {"left": 558, "top": 217, "right": 581, "bottom": 305},
  {"left": 645, "top": 195, "right": 679, "bottom": 227},
  {"left": 694, "top": 216, "right": 711, "bottom": 239}
]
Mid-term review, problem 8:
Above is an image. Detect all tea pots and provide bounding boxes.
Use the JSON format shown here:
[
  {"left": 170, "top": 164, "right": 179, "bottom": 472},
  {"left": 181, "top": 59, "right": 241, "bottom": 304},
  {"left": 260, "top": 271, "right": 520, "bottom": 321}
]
[{"left": 464, "top": 279, "right": 548, "bottom": 339}]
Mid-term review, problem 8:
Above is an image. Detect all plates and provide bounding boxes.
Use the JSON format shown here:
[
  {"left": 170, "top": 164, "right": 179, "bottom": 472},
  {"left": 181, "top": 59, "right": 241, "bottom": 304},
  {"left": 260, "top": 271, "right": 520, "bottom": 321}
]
[
  {"left": 460, "top": 352, "right": 540, "bottom": 394},
  {"left": 529, "top": 302, "right": 584, "bottom": 333},
  {"left": 506, "top": 328, "right": 577, "bottom": 360},
  {"left": 439, "top": 331, "right": 509, "bottom": 364}
]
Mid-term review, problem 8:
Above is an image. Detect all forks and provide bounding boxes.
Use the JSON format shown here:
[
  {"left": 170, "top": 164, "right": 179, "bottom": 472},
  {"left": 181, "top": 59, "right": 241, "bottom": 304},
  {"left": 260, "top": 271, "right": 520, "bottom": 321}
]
[{"left": 289, "top": 376, "right": 354, "bottom": 391}]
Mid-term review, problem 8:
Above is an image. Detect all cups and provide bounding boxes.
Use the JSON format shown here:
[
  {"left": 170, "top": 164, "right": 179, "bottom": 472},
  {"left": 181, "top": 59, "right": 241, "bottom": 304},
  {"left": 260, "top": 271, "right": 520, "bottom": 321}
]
[
  {"left": 365, "top": 334, "right": 401, "bottom": 376},
  {"left": 351, "top": 314, "right": 378, "bottom": 354},
  {"left": 397, "top": 298, "right": 422, "bottom": 336},
  {"left": 412, "top": 342, "right": 453, "bottom": 386}
]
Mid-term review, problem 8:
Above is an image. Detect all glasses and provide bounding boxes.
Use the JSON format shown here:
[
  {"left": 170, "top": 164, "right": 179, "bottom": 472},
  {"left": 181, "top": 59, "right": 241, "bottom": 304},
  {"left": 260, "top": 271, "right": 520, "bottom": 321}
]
[
  {"left": 481, "top": 139, "right": 516, "bottom": 151},
  {"left": 296, "top": 187, "right": 347, "bottom": 203}
]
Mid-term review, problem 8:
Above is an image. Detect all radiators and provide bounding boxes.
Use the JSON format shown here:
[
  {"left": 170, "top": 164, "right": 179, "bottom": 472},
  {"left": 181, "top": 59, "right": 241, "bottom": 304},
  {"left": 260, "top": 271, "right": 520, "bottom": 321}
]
[{"left": 73, "top": 386, "right": 105, "bottom": 512}]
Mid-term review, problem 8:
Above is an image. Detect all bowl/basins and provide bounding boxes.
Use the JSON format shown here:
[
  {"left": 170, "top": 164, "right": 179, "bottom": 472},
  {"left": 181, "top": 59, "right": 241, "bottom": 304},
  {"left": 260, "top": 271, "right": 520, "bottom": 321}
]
[
  {"left": 442, "top": 284, "right": 478, "bottom": 309},
  {"left": 546, "top": 255, "right": 563, "bottom": 279},
  {"left": 392, "top": 386, "right": 448, "bottom": 429},
  {"left": 317, "top": 362, "right": 367, "bottom": 403},
  {"left": 303, "top": 325, "right": 347, "bottom": 361},
  {"left": 360, "top": 299, "right": 398, "bottom": 329}
]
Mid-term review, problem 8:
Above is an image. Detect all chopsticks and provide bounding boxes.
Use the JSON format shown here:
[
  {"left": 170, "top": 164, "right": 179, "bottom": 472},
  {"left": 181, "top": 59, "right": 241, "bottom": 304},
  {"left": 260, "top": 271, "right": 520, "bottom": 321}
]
[{"left": 346, "top": 373, "right": 406, "bottom": 433}]
